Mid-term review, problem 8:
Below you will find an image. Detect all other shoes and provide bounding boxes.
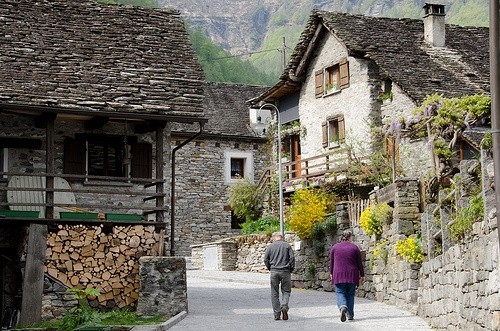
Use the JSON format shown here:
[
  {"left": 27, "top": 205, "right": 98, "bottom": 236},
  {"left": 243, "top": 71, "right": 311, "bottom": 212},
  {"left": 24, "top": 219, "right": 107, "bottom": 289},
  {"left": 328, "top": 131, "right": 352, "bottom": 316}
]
[
  {"left": 282, "top": 308, "right": 288, "bottom": 320},
  {"left": 348, "top": 318, "right": 353, "bottom": 320},
  {"left": 340, "top": 307, "right": 346, "bottom": 322}
]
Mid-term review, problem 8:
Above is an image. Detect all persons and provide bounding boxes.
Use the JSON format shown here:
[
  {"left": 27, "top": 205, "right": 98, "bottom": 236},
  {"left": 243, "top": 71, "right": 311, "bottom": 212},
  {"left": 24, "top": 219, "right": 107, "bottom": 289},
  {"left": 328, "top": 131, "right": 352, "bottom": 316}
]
[
  {"left": 263, "top": 232, "right": 296, "bottom": 320},
  {"left": 436, "top": 153, "right": 460, "bottom": 178},
  {"left": 330, "top": 230, "right": 365, "bottom": 322}
]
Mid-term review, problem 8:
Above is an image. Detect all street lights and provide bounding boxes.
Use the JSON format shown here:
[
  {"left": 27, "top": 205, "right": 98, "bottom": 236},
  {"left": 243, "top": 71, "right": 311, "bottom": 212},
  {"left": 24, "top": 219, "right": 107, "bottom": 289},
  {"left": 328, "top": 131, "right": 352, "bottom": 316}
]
[{"left": 258, "top": 104, "right": 283, "bottom": 238}]
